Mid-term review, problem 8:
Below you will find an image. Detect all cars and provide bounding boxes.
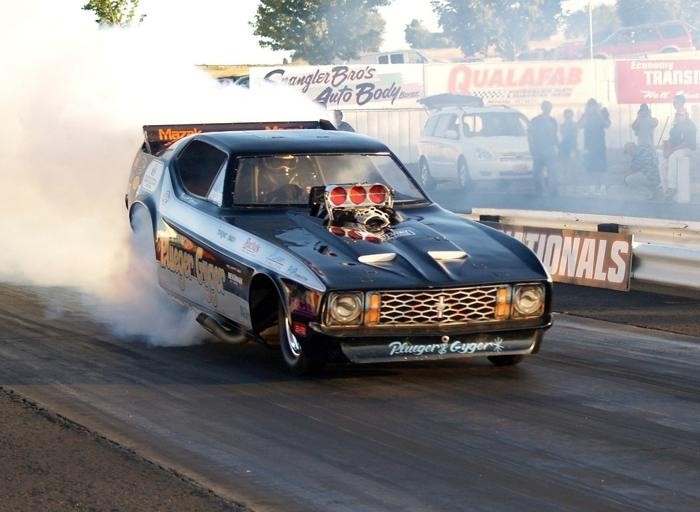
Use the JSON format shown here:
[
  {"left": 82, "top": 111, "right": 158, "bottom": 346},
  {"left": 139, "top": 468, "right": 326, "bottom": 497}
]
[
  {"left": 375, "top": 49, "right": 431, "bottom": 64},
  {"left": 125, "top": 121, "right": 553, "bottom": 377},
  {"left": 416, "top": 93, "right": 535, "bottom": 193},
  {"left": 588, "top": 21, "right": 693, "bottom": 59}
]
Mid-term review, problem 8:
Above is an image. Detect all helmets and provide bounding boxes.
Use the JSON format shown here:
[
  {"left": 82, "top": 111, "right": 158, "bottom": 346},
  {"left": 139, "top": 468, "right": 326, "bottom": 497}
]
[{"left": 262, "top": 155, "right": 298, "bottom": 185}]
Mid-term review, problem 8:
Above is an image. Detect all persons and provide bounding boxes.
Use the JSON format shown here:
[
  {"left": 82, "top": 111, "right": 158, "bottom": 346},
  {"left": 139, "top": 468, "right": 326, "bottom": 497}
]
[
  {"left": 663, "top": 94, "right": 689, "bottom": 157},
  {"left": 665, "top": 106, "right": 696, "bottom": 200},
  {"left": 527, "top": 100, "right": 559, "bottom": 197},
  {"left": 333, "top": 110, "right": 355, "bottom": 132},
  {"left": 578, "top": 98, "right": 610, "bottom": 196},
  {"left": 623, "top": 142, "right": 661, "bottom": 200},
  {"left": 631, "top": 104, "right": 658, "bottom": 146},
  {"left": 559, "top": 109, "right": 579, "bottom": 178}
]
